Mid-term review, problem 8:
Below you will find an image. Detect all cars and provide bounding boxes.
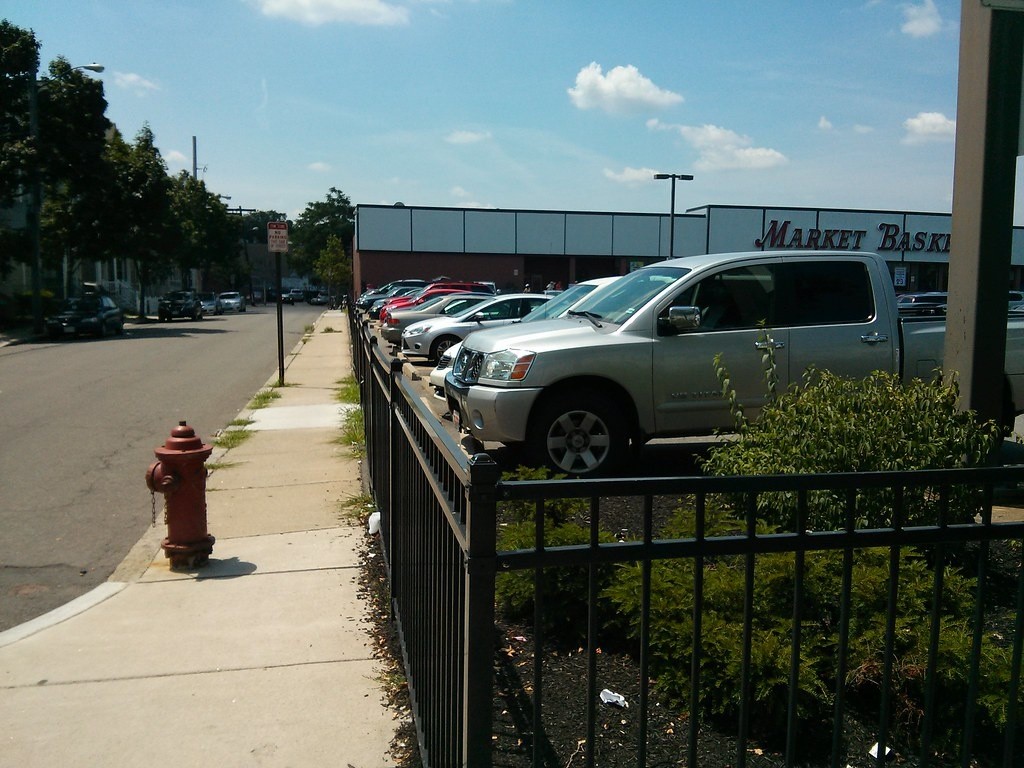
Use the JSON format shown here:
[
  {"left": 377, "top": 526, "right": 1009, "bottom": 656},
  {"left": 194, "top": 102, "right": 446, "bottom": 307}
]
[
  {"left": 156, "top": 290, "right": 203, "bottom": 322},
  {"left": 360, "top": 246, "right": 636, "bottom": 413},
  {"left": 197, "top": 291, "right": 222, "bottom": 316},
  {"left": 282, "top": 288, "right": 332, "bottom": 306},
  {"left": 219, "top": 291, "right": 246, "bottom": 314},
  {"left": 44, "top": 292, "right": 124, "bottom": 340},
  {"left": 895, "top": 291, "right": 1024, "bottom": 319}
]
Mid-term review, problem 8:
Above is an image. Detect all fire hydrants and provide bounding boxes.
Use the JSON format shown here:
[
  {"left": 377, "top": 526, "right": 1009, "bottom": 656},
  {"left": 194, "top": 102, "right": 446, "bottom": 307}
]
[{"left": 146, "top": 420, "right": 215, "bottom": 572}]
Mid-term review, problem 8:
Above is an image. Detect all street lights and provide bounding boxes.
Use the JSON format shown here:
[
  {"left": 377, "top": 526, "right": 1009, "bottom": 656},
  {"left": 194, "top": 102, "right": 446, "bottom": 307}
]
[
  {"left": 654, "top": 173, "right": 694, "bottom": 259},
  {"left": 23, "top": 59, "right": 105, "bottom": 337}
]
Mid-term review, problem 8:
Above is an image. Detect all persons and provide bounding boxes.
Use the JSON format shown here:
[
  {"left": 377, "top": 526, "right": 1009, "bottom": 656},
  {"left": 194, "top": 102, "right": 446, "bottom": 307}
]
[
  {"left": 523, "top": 284, "right": 530, "bottom": 293},
  {"left": 546, "top": 281, "right": 562, "bottom": 290}
]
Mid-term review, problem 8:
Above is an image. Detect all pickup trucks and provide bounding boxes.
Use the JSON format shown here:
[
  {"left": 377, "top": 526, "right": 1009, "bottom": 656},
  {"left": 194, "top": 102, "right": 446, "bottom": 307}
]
[{"left": 446, "top": 251, "right": 1024, "bottom": 479}]
